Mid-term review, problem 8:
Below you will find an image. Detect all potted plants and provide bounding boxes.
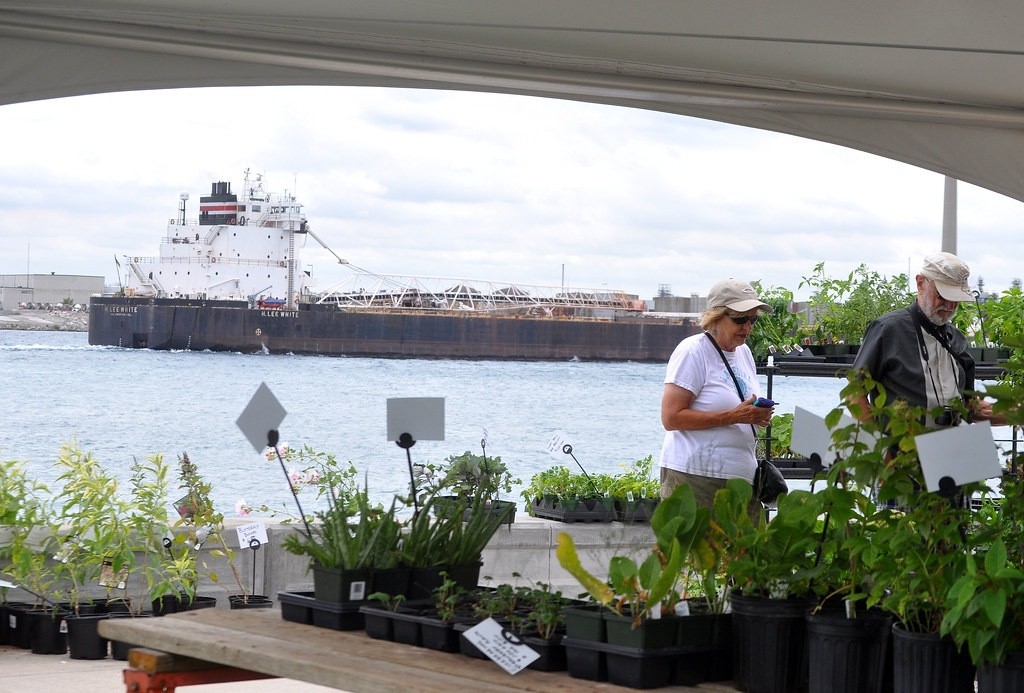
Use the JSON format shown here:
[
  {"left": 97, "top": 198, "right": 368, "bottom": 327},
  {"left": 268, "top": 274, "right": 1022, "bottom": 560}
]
[{"left": 0, "top": 262, "right": 1024, "bottom": 692}]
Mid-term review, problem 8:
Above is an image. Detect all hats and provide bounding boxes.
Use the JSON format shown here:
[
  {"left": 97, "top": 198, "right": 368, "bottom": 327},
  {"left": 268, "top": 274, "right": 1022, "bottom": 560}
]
[
  {"left": 920, "top": 251, "right": 976, "bottom": 302},
  {"left": 707, "top": 277, "right": 774, "bottom": 314}
]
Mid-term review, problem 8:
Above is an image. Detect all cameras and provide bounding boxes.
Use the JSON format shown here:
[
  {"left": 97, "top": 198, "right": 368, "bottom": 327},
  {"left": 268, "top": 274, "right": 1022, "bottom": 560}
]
[{"left": 934, "top": 405, "right": 961, "bottom": 427}]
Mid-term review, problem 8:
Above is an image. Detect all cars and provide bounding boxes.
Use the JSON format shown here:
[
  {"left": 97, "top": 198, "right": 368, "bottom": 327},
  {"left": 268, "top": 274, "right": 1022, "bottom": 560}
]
[{"left": 19, "top": 300, "right": 90, "bottom": 313}]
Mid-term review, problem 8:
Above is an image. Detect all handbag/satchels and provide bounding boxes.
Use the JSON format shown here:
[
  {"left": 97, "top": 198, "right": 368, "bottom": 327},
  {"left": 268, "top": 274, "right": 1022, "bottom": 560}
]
[{"left": 753, "top": 458, "right": 788, "bottom": 508}]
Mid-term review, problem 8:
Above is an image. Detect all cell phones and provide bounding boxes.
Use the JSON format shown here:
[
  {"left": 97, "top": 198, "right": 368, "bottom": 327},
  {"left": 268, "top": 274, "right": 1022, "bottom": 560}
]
[{"left": 752, "top": 397, "right": 774, "bottom": 409}]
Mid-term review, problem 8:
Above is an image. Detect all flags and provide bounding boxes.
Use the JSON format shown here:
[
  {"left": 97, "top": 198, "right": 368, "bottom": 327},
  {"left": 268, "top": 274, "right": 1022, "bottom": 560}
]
[{"left": 115, "top": 258, "right": 121, "bottom": 267}]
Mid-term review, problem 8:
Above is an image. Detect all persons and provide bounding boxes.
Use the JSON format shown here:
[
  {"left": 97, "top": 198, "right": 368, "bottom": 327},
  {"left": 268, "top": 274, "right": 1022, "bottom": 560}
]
[
  {"left": 845, "top": 252, "right": 994, "bottom": 528},
  {"left": 658, "top": 278, "right": 775, "bottom": 583}
]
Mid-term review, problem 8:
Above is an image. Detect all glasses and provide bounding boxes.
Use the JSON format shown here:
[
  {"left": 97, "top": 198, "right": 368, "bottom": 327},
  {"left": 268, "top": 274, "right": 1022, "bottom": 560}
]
[
  {"left": 924, "top": 277, "right": 948, "bottom": 301},
  {"left": 724, "top": 311, "right": 760, "bottom": 324}
]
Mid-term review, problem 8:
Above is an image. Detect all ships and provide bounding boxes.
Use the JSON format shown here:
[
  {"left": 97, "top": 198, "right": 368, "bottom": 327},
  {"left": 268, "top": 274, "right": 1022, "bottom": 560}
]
[{"left": 125, "top": 168, "right": 312, "bottom": 301}]
